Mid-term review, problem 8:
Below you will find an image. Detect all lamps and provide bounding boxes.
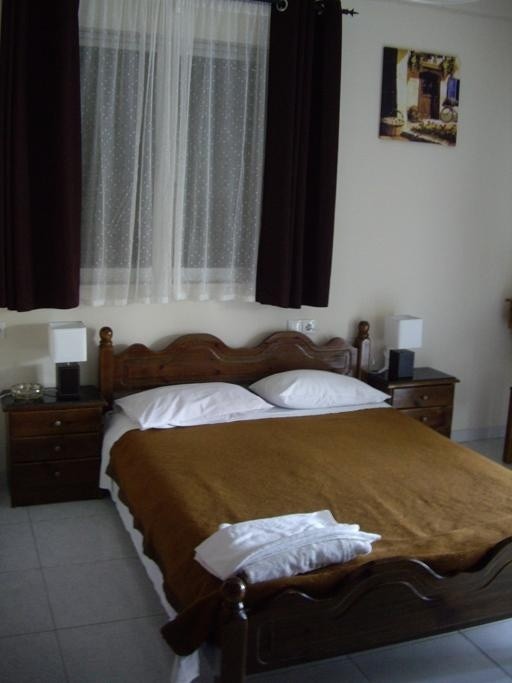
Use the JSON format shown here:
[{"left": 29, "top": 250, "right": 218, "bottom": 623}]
[
  {"left": 46, "top": 320, "right": 88, "bottom": 400},
  {"left": 383, "top": 314, "right": 422, "bottom": 380}
]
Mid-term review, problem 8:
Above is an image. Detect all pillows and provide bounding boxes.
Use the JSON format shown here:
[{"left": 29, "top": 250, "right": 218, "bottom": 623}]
[{"left": 113, "top": 368, "right": 392, "bottom": 431}]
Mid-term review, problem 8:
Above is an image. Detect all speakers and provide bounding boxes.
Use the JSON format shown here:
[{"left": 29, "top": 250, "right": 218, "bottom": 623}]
[
  {"left": 55, "top": 363, "right": 80, "bottom": 399},
  {"left": 389, "top": 348, "right": 415, "bottom": 378}
]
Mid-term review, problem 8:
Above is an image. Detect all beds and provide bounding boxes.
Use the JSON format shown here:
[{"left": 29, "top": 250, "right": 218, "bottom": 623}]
[{"left": 95, "top": 321, "right": 512, "bottom": 683}]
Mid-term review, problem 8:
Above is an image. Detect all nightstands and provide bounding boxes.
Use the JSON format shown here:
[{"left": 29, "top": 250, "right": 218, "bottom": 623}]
[
  {"left": 373, "top": 366, "right": 461, "bottom": 441},
  {"left": 1, "top": 386, "right": 111, "bottom": 509}
]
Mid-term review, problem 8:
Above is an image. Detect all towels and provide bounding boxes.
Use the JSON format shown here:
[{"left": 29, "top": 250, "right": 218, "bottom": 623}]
[{"left": 191, "top": 510, "right": 381, "bottom": 586}]
